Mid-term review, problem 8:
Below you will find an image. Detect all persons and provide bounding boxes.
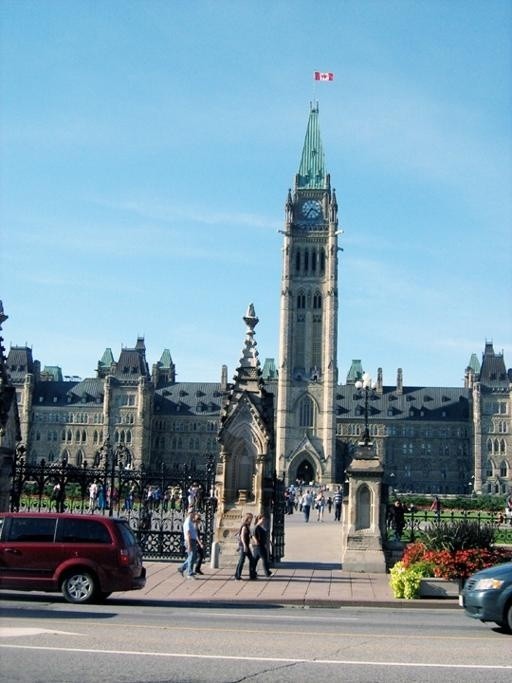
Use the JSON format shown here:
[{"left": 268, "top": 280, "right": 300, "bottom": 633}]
[
  {"left": 234, "top": 513, "right": 258, "bottom": 582},
  {"left": 89, "top": 477, "right": 204, "bottom": 580},
  {"left": 386, "top": 499, "right": 415, "bottom": 542},
  {"left": 249, "top": 513, "right": 272, "bottom": 580},
  {"left": 53, "top": 479, "right": 64, "bottom": 513},
  {"left": 505, "top": 493, "right": 512, "bottom": 524},
  {"left": 283, "top": 477, "right": 343, "bottom": 523},
  {"left": 430, "top": 496, "right": 440, "bottom": 522}
]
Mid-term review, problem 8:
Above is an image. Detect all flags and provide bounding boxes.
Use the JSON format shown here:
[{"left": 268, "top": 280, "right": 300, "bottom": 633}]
[{"left": 314, "top": 72, "right": 334, "bottom": 81}]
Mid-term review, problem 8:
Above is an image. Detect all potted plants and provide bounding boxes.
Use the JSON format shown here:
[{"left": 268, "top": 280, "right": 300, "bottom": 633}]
[{"left": 414, "top": 518, "right": 495, "bottom": 597}]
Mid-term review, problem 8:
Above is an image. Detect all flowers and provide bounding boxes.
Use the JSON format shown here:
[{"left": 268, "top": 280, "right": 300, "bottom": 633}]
[{"left": 388, "top": 542, "right": 511, "bottom": 601}]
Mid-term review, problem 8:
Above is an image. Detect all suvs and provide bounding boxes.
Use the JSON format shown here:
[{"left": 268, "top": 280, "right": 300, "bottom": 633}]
[{"left": 0, "top": 510, "right": 151, "bottom": 605}]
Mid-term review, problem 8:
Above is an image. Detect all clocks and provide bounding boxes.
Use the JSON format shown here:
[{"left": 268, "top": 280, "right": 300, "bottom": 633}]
[{"left": 302, "top": 199, "right": 323, "bottom": 219}]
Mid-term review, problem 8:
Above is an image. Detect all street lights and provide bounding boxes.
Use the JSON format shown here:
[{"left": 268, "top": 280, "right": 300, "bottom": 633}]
[{"left": 352, "top": 372, "right": 381, "bottom": 446}]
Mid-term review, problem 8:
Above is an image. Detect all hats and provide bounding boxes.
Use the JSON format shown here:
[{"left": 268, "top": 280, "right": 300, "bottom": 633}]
[{"left": 187, "top": 507, "right": 198, "bottom": 513}]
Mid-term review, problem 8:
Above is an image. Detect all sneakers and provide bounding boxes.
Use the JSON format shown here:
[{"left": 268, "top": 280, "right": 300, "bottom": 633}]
[
  {"left": 196, "top": 571, "right": 204, "bottom": 575},
  {"left": 177, "top": 567, "right": 184, "bottom": 577},
  {"left": 187, "top": 575, "right": 199, "bottom": 581}
]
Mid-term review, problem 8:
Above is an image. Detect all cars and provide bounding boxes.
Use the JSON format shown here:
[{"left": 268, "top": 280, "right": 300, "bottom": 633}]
[{"left": 457, "top": 559, "right": 512, "bottom": 633}]
